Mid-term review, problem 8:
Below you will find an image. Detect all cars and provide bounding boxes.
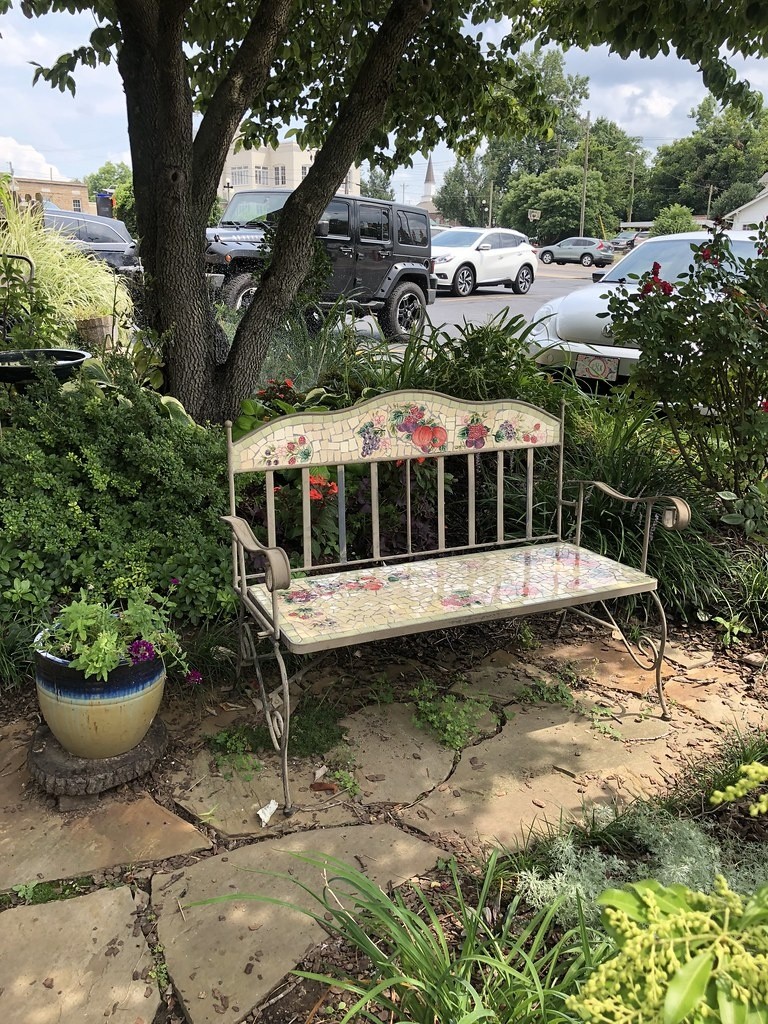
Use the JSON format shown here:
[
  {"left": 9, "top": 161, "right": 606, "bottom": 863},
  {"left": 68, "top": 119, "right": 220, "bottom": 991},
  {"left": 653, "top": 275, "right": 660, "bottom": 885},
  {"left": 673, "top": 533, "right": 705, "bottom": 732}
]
[
  {"left": 539, "top": 237, "right": 614, "bottom": 268},
  {"left": 43, "top": 209, "right": 139, "bottom": 274},
  {"left": 403, "top": 226, "right": 450, "bottom": 238},
  {"left": 519, "top": 231, "right": 768, "bottom": 399},
  {"left": 634, "top": 232, "right": 651, "bottom": 248},
  {"left": 610, "top": 230, "right": 639, "bottom": 250},
  {"left": 431, "top": 226, "right": 538, "bottom": 297}
]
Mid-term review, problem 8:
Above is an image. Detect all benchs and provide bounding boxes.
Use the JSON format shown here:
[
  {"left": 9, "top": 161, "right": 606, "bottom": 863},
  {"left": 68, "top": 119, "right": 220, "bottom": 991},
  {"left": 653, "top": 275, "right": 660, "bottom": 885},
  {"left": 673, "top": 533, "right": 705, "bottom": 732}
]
[{"left": 219, "top": 388, "right": 691, "bottom": 818}]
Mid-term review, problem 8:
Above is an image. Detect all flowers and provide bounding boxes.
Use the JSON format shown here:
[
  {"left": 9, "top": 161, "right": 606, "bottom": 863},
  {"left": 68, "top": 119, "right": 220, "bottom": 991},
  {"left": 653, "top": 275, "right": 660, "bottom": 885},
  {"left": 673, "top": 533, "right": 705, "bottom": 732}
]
[{"left": 29, "top": 576, "right": 203, "bottom": 684}]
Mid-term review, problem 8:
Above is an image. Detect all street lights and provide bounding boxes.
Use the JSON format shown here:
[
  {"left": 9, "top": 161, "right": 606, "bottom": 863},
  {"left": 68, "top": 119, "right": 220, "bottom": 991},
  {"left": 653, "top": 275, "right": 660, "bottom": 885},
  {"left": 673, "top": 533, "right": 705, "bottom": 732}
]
[
  {"left": 625, "top": 149, "right": 642, "bottom": 221},
  {"left": 480, "top": 199, "right": 489, "bottom": 227},
  {"left": 223, "top": 178, "right": 234, "bottom": 203}
]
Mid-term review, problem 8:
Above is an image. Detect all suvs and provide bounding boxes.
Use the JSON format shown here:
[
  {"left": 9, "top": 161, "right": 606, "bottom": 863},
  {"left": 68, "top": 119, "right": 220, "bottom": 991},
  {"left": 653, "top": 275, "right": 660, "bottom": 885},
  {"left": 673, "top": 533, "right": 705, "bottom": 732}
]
[{"left": 125, "top": 189, "right": 438, "bottom": 344}]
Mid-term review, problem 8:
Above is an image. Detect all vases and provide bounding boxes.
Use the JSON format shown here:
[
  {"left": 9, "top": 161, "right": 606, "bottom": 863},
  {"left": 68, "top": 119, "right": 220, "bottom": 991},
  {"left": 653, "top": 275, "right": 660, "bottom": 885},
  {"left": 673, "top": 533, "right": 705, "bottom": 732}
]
[
  {"left": 79, "top": 315, "right": 118, "bottom": 352},
  {"left": 0, "top": 347, "right": 92, "bottom": 393},
  {"left": 33, "top": 612, "right": 167, "bottom": 757}
]
